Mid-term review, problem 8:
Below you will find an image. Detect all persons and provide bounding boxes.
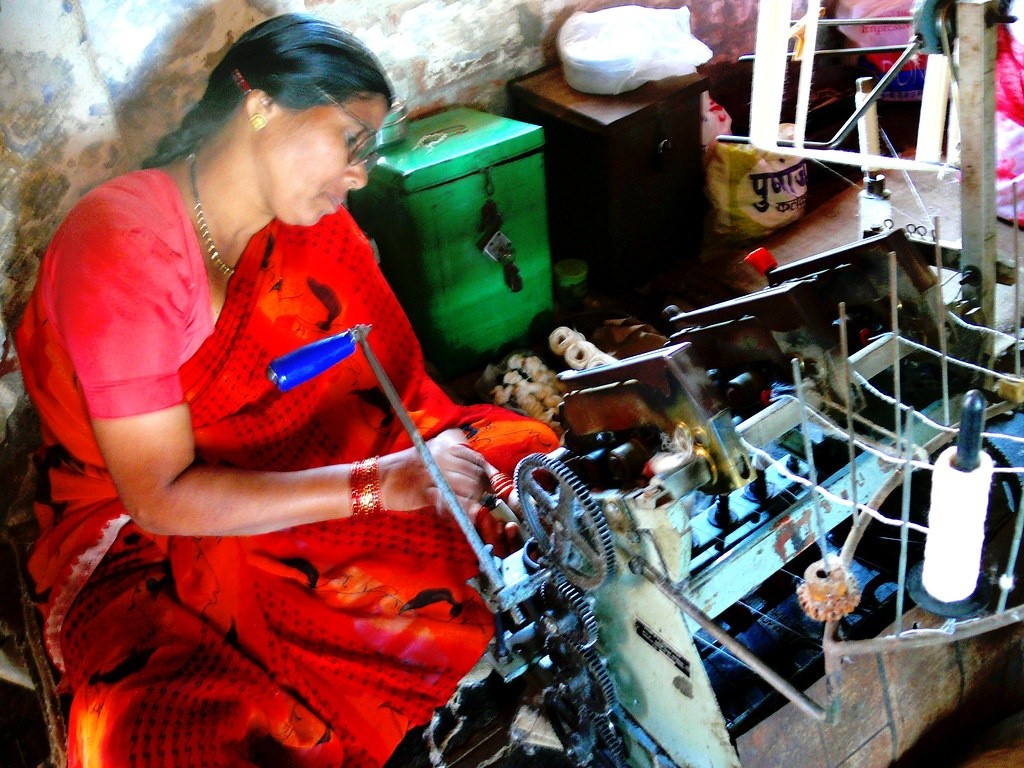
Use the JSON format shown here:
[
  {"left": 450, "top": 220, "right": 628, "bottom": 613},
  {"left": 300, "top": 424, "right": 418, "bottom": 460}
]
[{"left": 11, "top": 13, "right": 560, "bottom": 768}]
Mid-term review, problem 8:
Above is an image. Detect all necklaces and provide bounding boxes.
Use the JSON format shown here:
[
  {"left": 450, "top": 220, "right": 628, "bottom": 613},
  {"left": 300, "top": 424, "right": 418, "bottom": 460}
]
[{"left": 186, "top": 136, "right": 236, "bottom": 282}]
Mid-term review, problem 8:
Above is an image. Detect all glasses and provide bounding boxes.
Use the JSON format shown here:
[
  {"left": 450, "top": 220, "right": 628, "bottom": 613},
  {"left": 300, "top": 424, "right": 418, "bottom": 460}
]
[{"left": 314, "top": 84, "right": 381, "bottom": 174}]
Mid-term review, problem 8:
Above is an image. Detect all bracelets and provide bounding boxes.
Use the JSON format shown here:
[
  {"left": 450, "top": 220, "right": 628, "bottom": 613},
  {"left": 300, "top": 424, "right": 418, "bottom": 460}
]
[{"left": 348, "top": 455, "right": 387, "bottom": 520}]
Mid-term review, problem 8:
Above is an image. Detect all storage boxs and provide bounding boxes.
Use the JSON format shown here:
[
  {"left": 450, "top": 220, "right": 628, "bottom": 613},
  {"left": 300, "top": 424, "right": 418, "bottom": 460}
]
[
  {"left": 512, "top": 64, "right": 715, "bottom": 290},
  {"left": 346, "top": 106, "right": 556, "bottom": 374}
]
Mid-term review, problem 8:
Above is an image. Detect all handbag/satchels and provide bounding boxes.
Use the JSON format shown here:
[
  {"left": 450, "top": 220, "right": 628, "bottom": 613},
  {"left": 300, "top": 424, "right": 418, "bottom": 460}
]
[
  {"left": 703, "top": 122, "right": 809, "bottom": 247},
  {"left": 702, "top": 90, "right": 733, "bottom": 154}
]
